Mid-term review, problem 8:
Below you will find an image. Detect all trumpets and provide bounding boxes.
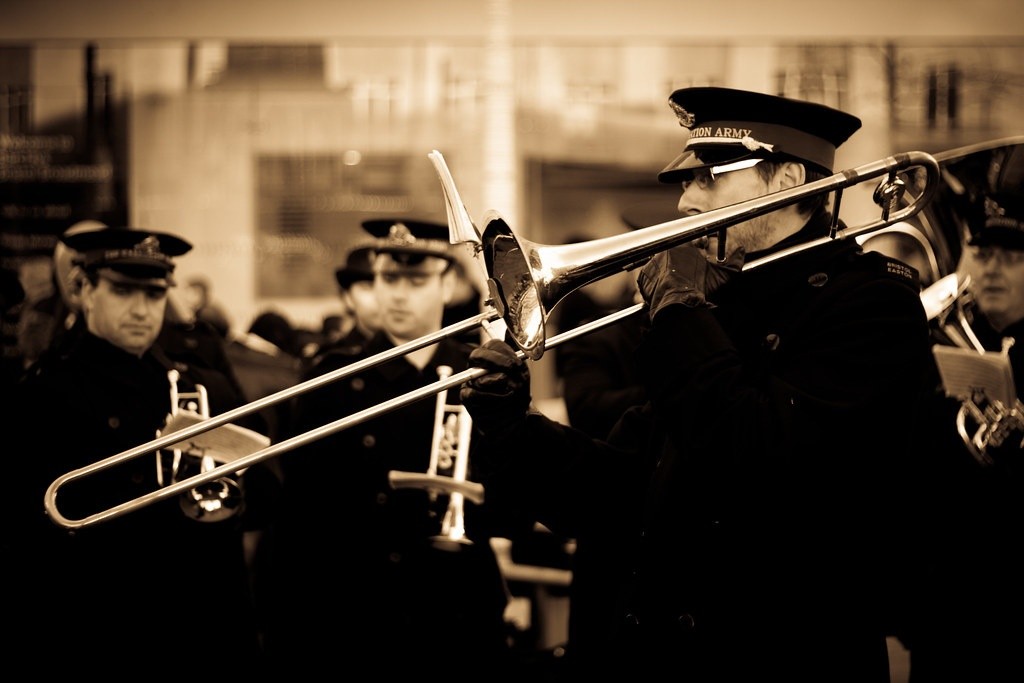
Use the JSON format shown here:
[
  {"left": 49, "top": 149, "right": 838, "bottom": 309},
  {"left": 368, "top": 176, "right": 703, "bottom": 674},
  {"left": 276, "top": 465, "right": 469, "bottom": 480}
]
[
  {"left": 155, "top": 368, "right": 244, "bottom": 524},
  {"left": 422, "top": 365, "right": 482, "bottom": 551}
]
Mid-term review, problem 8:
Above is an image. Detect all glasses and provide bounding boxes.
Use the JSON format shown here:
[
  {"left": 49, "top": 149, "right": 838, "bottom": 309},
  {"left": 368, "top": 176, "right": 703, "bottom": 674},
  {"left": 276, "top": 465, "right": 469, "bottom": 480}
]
[{"left": 680, "top": 157, "right": 763, "bottom": 190}]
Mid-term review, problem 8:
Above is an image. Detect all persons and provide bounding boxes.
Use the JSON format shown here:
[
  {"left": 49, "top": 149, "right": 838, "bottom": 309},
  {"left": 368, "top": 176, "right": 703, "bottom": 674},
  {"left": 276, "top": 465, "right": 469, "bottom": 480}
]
[{"left": 1, "top": 86, "right": 1024, "bottom": 683}]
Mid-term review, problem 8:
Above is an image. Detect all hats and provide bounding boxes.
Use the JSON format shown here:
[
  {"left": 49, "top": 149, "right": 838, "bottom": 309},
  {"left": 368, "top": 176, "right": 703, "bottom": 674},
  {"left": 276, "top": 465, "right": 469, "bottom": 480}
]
[
  {"left": 958, "top": 193, "right": 1024, "bottom": 250},
  {"left": 655, "top": 86, "right": 862, "bottom": 182},
  {"left": 361, "top": 217, "right": 451, "bottom": 258},
  {"left": 331, "top": 247, "right": 377, "bottom": 288},
  {"left": 62, "top": 228, "right": 193, "bottom": 289},
  {"left": 247, "top": 310, "right": 300, "bottom": 357}
]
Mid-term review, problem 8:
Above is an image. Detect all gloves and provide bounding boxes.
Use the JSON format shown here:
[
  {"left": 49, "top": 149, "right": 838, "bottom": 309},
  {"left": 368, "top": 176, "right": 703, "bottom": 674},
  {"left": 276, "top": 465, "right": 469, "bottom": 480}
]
[
  {"left": 641, "top": 240, "right": 746, "bottom": 319},
  {"left": 465, "top": 338, "right": 531, "bottom": 409}
]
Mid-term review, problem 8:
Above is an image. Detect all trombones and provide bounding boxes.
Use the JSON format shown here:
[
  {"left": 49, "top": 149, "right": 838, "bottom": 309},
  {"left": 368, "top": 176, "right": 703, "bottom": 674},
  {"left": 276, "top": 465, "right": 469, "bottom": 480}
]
[{"left": 43, "top": 149, "right": 942, "bottom": 528}]
